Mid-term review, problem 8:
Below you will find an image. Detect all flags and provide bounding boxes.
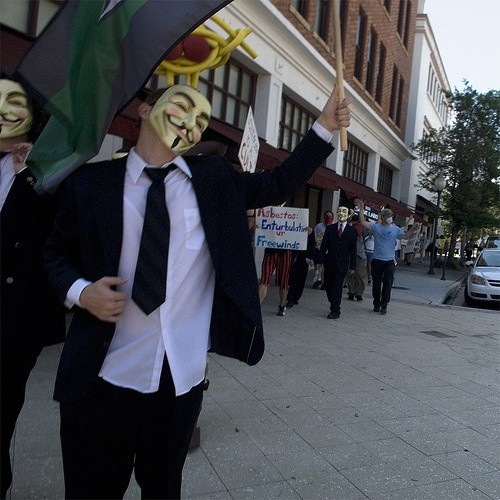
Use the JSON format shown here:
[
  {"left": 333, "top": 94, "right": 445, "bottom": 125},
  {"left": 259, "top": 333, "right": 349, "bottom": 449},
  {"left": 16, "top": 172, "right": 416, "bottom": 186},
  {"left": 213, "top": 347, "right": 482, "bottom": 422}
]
[{"left": 16, "top": 0, "right": 232, "bottom": 190}]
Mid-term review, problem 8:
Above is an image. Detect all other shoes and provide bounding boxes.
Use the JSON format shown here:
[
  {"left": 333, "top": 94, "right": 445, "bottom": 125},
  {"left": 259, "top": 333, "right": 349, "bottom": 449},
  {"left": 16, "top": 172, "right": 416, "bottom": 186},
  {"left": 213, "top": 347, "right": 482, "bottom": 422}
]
[
  {"left": 348, "top": 294, "right": 363, "bottom": 301},
  {"left": 312, "top": 280, "right": 325, "bottom": 290},
  {"left": 374, "top": 305, "right": 387, "bottom": 315},
  {"left": 277, "top": 304, "right": 287, "bottom": 316},
  {"left": 285, "top": 301, "right": 298, "bottom": 307},
  {"left": 327, "top": 304, "right": 341, "bottom": 319}
]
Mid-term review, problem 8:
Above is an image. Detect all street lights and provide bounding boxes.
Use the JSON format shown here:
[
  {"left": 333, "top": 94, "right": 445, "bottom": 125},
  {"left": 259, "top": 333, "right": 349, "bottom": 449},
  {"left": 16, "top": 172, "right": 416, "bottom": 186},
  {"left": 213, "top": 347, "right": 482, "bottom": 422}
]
[{"left": 426, "top": 171, "right": 447, "bottom": 275}]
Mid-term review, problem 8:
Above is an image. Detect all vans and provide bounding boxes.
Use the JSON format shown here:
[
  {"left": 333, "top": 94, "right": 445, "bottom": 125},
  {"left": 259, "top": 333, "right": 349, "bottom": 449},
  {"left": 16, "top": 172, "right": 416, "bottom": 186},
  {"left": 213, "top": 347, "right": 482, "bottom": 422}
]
[{"left": 472, "top": 235, "right": 500, "bottom": 258}]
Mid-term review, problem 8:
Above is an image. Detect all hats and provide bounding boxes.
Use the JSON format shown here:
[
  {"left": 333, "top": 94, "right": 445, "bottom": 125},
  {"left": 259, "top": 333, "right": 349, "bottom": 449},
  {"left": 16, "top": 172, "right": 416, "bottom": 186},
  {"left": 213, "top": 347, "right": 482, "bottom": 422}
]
[{"left": 381, "top": 209, "right": 393, "bottom": 218}]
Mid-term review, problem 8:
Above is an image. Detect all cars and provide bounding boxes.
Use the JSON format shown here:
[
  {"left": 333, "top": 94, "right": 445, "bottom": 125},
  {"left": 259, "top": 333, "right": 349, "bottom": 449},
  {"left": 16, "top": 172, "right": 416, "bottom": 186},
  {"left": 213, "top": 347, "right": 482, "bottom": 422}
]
[{"left": 463, "top": 248, "right": 500, "bottom": 308}]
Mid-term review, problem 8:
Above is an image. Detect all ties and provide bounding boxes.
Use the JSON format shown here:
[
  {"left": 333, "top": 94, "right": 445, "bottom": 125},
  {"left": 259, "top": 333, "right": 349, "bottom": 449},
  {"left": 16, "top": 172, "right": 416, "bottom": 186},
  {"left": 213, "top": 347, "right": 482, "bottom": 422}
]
[
  {"left": 131, "top": 162, "right": 178, "bottom": 317},
  {"left": 338, "top": 224, "right": 343, "bottom": 238}
]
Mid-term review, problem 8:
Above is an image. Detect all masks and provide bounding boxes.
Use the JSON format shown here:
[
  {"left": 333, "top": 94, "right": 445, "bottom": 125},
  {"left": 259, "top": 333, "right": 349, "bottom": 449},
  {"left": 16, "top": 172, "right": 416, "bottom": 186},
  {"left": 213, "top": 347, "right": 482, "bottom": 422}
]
[{"left": 385, "top": 217, "right": 392, "bottom": 224}]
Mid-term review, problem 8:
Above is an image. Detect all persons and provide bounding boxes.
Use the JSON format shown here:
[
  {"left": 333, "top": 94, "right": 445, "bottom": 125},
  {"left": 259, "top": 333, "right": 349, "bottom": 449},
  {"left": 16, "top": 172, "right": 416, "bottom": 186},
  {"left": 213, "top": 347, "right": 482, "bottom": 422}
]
[
  {"left": 285, "top": 227, "right": 316, "bottom": 308},
  {"left": 250, "top": 224, "right": 293, "bottom": 317},
  {"left": 465, "top": 238, "right": 475, "bottom": 261},
  {"left": 312, "top": 211, "right": 335, "bottom": 291},
  {"left": 43, "top": 81, "right": 351, "bottom": 500},
  {"left": 353, "top": 197, "right": 420, "bottom": 314},
  {"left": 365, "top": 212, "right": 448, "bottom": 285},
  {"left": 0, "top": 73, "right": 43, "bottom": 500},
  {"left": 316, "top": 206, "right": 358, "bottom": 319},
  {"left": 346, "top": 211, "right": 366, "bottom": 302}
]
